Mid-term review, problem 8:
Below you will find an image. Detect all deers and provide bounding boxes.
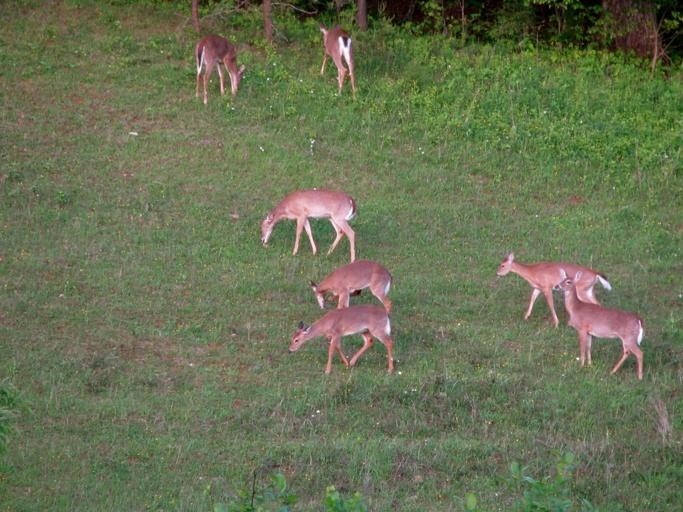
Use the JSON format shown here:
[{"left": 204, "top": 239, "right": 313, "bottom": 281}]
[
  {"left": 307, "top": 260, "right": 393, "bottom": 318},
  {"left": 496, "top": 252, "right": 613, "bottom": 330},
  {"left": 552, "top": 268, "right": 645, "bottom": 381},
  {"left": 287, "top": 303, "right": 394, "bottom": 376},
  {"left": 194, "top": 34, "right": 246, "bottom": 105},
  {"left": 319, "top": 22, "right": 355, "bottom": 95},
  {"left": 260, "top": 189, "right": 356, "bottom": 264}
]
